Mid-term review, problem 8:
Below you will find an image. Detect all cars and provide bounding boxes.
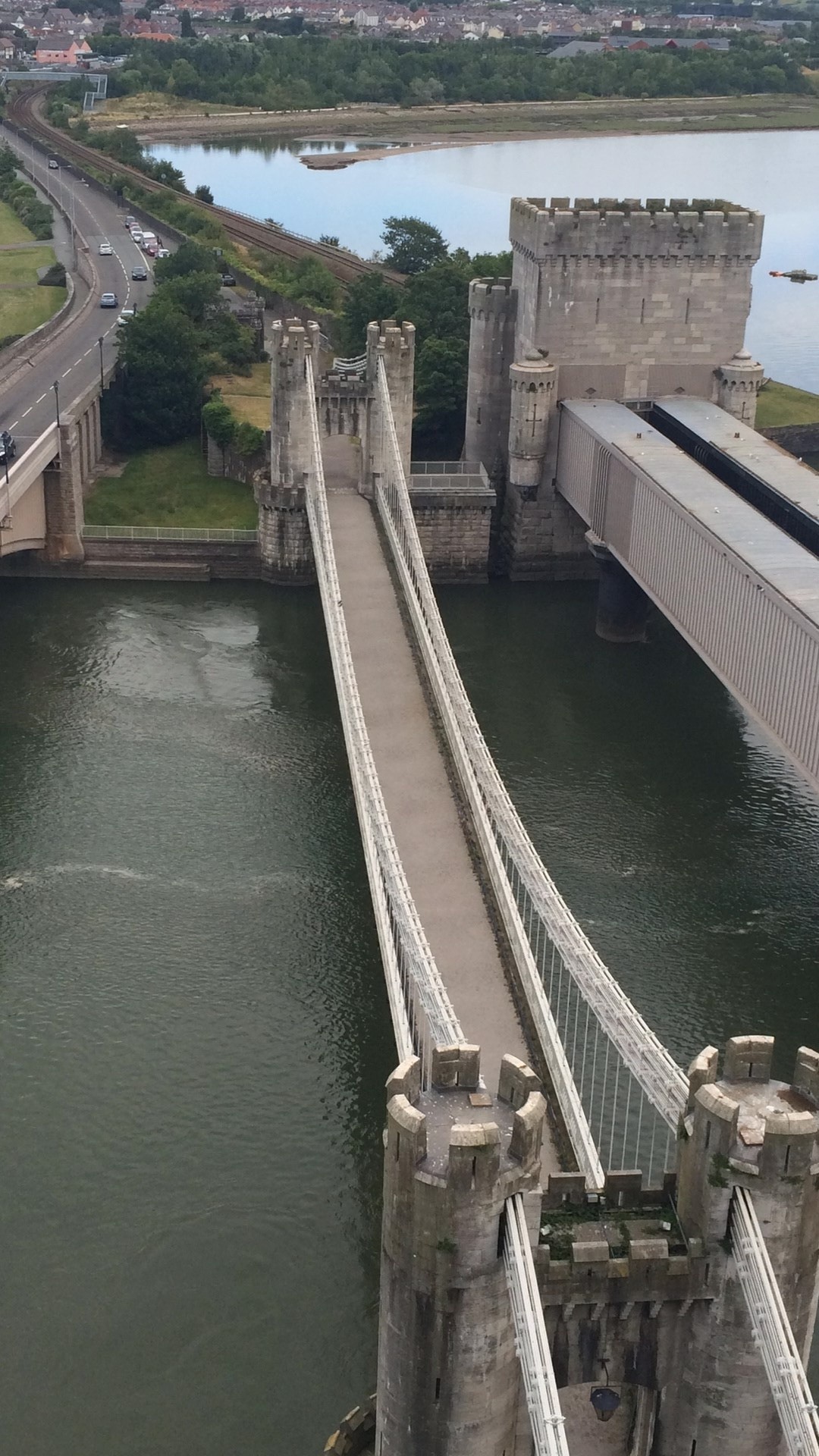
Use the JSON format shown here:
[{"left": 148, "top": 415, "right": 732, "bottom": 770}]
[
  {"left": 118, "top": 310, "right": 137, "bottom": 327},
  {"left": 131, "top": 226, "right": 142, "bottom": 236},
  {"left": 125, "top": 216, "right": 135, "bottom": 227},
  {"left": 148, "top": 244, "right": 160, "bottom": 256},
  {"left": 49, "top": 160, "right": 58, "bottom": 169},
  {"left": 220, "top": 273, "right": 236, "bottom": 287},
  {"left": 131, "top": 267, "right": 148, "bottom": 281},
  {"left": 98, "top": 244, "right": 112, "bottom": 255},
  {"left": 158, "top": 248, "right": 170, "bottom": 259},
  {"left": 129, "top": 222, "right": 140, "bottom": 232},
  {"left": 100, "top": 293, "right": 118, "bottom": 307},
  {"left": 133, "top": 232, "right": 143, "bottom": 242},
  {"left": 0, "top": 430, "right": 16, "bottom": 466}
]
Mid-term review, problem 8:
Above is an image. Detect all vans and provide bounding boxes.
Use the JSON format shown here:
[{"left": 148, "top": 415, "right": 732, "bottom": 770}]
[{"left": 141, "top": 231, "right": 157, "bottom": 250}]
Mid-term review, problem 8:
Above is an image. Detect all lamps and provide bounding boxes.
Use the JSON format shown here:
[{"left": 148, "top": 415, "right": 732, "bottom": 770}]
[{"left": 589, "top": 1359, "right": 622, "bottom": 1422}]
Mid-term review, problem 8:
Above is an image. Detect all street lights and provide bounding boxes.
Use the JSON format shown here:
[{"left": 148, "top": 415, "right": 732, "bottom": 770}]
[
  {"left": 16, "top": 128, "right": 27, "bottom": 159},
  {"left": 1, "top": 119, "right": 12, "bottom": 141},
  {"left": 70, "top": 179, "right": 89, "bottom": 273},
  {"left": 59, "top": 165, "right": 72, "bottom": 213},
  {"left": 31, "top": 139, "right": 44, "bottom": 182},
  {"left": 46, "top": 152, "right": 59, "bottom": 200}
]
